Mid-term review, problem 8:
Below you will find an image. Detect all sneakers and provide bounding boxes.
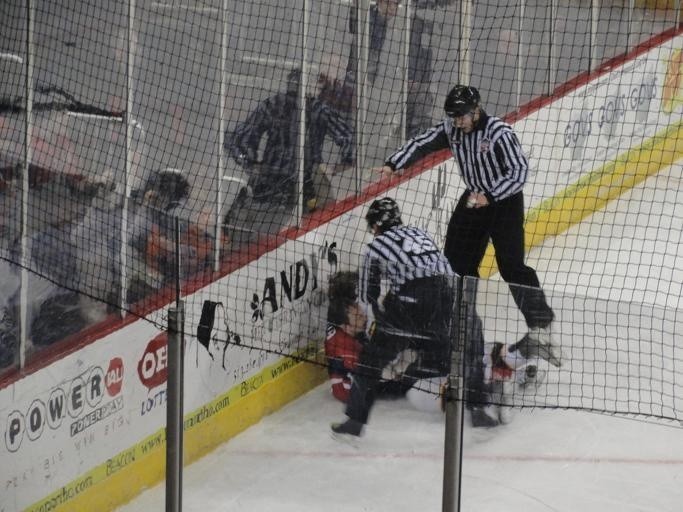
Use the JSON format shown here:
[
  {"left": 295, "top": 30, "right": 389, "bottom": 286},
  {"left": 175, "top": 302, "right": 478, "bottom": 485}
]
[{"left": 332, "top": 420, "right": 363, "bottom": 435}]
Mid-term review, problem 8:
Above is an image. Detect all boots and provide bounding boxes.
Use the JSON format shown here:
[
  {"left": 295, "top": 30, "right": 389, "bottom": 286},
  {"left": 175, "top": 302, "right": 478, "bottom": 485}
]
[{"left": 509, "top": 340, "right": 559, "bottom": 368}]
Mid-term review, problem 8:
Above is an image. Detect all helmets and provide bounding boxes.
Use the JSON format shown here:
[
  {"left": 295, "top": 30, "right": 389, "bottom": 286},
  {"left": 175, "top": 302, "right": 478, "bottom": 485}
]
[
  {"left": 146, "top": 168, "right": 190, "bottom": 212},
  {"left": 444, "top": 85, "right": 482, "bottom": 118},
  {"left": 366, "top": 197, "right": 400, "bottom": 231}
]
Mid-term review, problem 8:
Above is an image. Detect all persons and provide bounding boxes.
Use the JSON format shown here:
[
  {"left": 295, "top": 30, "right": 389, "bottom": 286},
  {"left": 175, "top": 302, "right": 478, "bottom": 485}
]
[
  {"left": 341, "top": 0, "right": 450, "bottom": 143},
  {"left": 375, "top": 84, "right": 555, "bottom": 348},
  {"left": 325, "top": 196, "right": 562, "bottom": 440},
  {"left": 1, "top": 69, "right": 356, "bottom": 373}
]
